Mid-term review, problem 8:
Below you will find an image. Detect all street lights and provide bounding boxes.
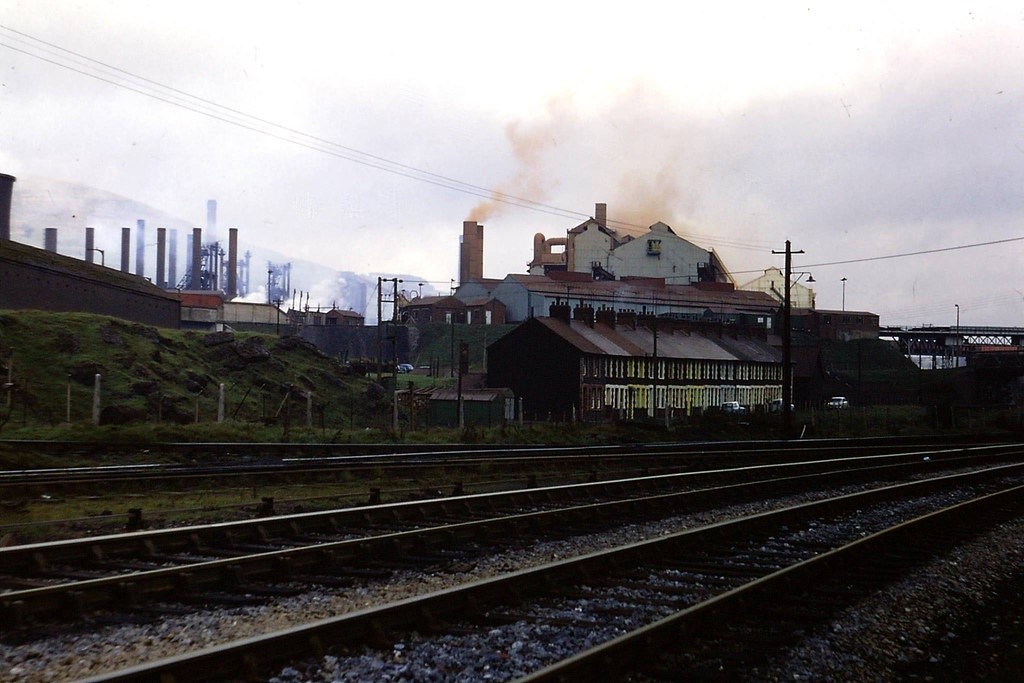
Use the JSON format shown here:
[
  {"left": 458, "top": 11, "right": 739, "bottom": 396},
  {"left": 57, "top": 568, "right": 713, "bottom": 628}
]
[
  {"left": 781, "top": 272, "right": 817, "bottom": 433},
  {"left": 954, "top": 304, "right": 959, "bottom": 368},
  {"left": 841, "top": 277, "right": 848, "bottom": 312}
]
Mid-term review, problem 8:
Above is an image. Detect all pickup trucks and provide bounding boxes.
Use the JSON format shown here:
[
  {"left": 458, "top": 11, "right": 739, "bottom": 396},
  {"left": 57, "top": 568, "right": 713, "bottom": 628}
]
[{"left": 720, "top": 400, "right": 746, "bottom": 417}]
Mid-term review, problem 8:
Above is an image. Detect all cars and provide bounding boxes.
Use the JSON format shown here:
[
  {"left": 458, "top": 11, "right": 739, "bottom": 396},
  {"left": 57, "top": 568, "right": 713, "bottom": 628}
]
[
  {"left": 771, "top": 398, "right": 799, "bottom": 414},
  {"left": 396, "top": 363, "right": 414, "bottom": 374},
  {"left": 827, "top": 397, "right": 848, "bottom": 410}
]
